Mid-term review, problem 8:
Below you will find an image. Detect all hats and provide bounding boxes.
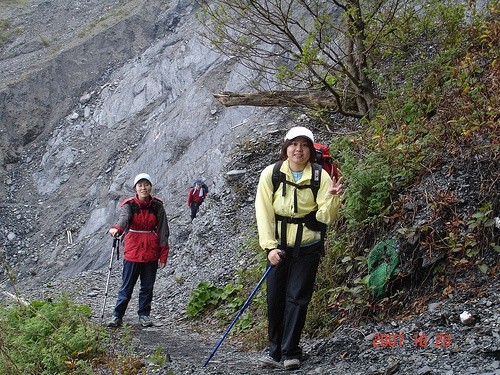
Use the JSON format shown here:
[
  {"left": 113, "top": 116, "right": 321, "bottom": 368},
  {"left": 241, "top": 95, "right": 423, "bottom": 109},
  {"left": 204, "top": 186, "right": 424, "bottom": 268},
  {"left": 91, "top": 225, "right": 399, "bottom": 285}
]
[
  {"left": 284, "top": 127, "right": 315, "bottom": 145},
  {"left": 134, "top": 173, "right": 152, "bottom": 186},
  {"left": 196, "top": 180, "right": 201, "bottom": 185}
]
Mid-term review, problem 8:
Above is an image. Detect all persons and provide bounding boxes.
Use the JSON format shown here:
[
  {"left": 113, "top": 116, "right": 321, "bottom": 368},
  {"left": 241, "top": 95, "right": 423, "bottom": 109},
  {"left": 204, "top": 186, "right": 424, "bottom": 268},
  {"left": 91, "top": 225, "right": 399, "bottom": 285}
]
[
  {"left": 105, "top": 173, "right": 171, "bottom": 327},
  {"left": 187, "top": 180, "right": 208, "bottom": 222},
  {"left": 255, "top": 126, "right": 342, "bottom": 368}
]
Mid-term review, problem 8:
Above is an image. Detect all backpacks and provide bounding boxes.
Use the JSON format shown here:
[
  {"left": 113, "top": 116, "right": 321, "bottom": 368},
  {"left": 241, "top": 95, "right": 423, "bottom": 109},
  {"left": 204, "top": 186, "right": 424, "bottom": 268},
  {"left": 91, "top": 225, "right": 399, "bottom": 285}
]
[{"left": 272, "top": 143, "right": 339, "bottom": 205}]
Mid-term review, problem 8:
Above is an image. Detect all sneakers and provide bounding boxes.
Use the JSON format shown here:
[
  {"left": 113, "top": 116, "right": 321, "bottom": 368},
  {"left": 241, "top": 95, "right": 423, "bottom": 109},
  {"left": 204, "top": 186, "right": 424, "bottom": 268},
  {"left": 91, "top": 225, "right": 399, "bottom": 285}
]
[
  {"left": 261, "top": 356, "right": 277, "bottom": 368},
  {"left": 284, "top": 358, "right": 300, "bottom": 370},
  {"left": 139, "top": 315, "right": 152, "bottom": 326},
  {"left": 107, "top": 316, "right": 121, "bottom": 326}
]
